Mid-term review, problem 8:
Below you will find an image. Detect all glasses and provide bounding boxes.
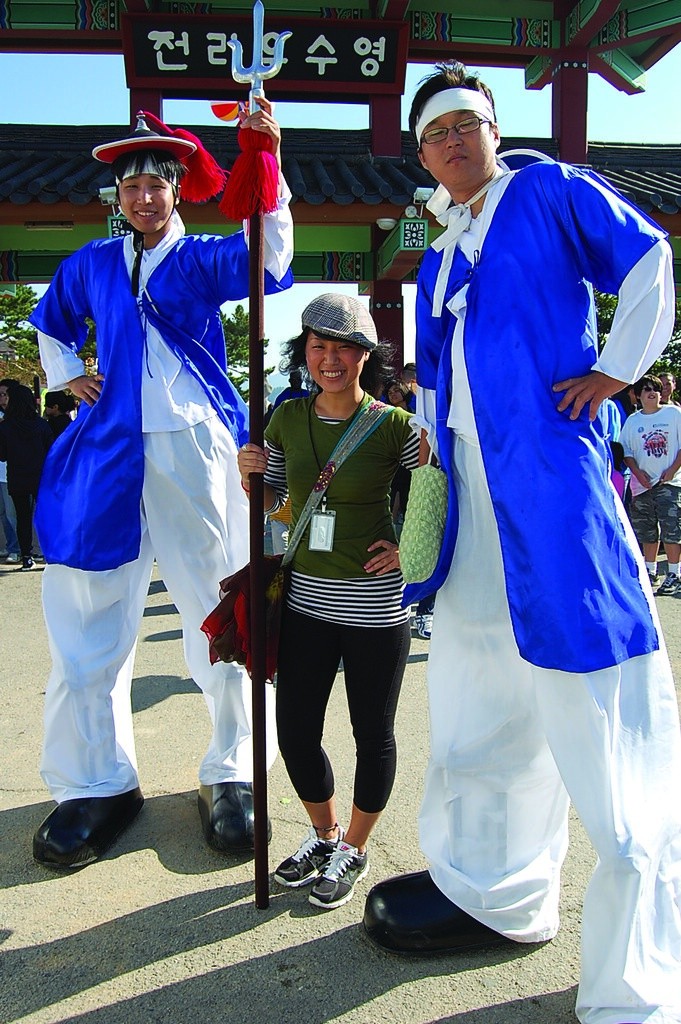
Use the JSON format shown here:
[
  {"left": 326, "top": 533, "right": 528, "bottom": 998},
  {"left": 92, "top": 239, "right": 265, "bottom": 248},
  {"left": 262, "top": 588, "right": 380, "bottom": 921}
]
[
  {"left": 641, "top": 387, "right": 660, "bottom": 392},
  {"left": 420, "top": 117, "right": 492, "bottom": 144}
]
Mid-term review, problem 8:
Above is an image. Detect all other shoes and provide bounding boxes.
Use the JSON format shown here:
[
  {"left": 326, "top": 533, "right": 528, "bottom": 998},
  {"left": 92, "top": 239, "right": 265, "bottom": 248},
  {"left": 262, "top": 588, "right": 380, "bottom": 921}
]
[
  {"left": 0, "top": 551, "right": 8, "bottom": 556},
  {"left": 32, "top": 786, "right": 146, "bottom": 869},
  {"left": 7, "top": 553, "right": 20, "bottom": 562},
  {"left": 361, "top": 867, "right": 512, "bottom": 956},
  {"left": 199, "top": 782, "right": 273, "bottom": 856}
]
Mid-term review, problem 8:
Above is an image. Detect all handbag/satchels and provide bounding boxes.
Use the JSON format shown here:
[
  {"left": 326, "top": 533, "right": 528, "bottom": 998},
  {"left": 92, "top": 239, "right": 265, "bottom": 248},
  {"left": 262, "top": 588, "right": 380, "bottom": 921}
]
[{"left": 399, "top": 462, "right": 449, "bottom": 585}]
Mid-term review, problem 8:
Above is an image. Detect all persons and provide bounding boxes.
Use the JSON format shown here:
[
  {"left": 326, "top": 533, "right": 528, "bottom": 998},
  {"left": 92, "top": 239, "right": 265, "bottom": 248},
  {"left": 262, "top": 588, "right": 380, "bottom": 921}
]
[
  {"left": 22, "top": 95, "right": 296, "bottom": 873},
  {"left": 237, "top": 292, "right": 435, "bottom": 911},
  {"left": 606, "top": 373, "right": 681, "bottom": 598},
  {"left": 263, "top": 362, "right": 434, "bottom": 640},
  {"left": 364, "top": 59, "right": 681, "bottom": 1024},
  {"left": 0, "top": 377, "right": 77, "bottom": 572}
]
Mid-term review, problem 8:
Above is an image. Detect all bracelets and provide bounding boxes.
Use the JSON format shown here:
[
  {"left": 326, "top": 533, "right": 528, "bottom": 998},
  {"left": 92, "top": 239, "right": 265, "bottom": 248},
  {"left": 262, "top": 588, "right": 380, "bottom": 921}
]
[{"left": 240, "top": 480, "right": 250, "bottom": 493}]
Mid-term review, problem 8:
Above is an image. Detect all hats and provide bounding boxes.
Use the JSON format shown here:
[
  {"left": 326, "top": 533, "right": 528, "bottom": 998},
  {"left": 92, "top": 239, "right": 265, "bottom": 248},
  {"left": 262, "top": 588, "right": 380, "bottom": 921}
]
[
  {"left": 91, "top": 112, "right": 228, "bottom": 202},
  {"left": 301, "top": 294, "right": 377, "bottom": 351}
]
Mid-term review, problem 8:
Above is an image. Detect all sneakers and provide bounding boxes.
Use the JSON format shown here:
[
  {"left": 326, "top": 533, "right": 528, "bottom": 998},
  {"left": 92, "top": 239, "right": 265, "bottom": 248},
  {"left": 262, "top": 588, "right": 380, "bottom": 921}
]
[
  {"left": 22, "top": 557, "right": 37, "bottom": 571},
  {"left": 657, "top": 572, "right": 681, "bottom": 595},
  {"left": 274, "top": 824, "right": 347, "bottom": 889},
  {"left": 647, "top": 568, "right": 660, "bottom": 587},
  {"left": 308, "top": 844, "right": 370, "bottom": 909}
]
